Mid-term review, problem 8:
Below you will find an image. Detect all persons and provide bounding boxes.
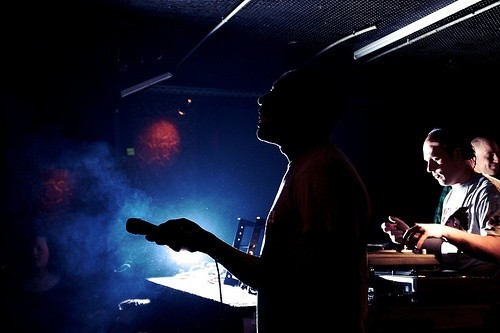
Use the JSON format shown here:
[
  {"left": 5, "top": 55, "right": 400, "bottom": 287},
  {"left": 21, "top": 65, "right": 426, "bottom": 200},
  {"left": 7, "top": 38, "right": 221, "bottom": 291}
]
[
  {"left": 465, "top": 136, "right": 498, "bottom": 194},
  {"left": 143, "top": 70, "right": 376, "bottom": 333},
  {"left": 381, "top": 125, "right": 500, "bottom": 277},
  {"left": 16, "top": 229, "right": 80, "bottom": 333}
]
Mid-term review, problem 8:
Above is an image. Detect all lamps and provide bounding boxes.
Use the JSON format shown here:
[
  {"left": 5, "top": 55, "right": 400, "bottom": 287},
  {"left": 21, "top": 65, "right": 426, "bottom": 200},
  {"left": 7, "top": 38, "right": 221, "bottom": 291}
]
[{"left": 176, "top": 92, "right": 192, "bottom": 116}]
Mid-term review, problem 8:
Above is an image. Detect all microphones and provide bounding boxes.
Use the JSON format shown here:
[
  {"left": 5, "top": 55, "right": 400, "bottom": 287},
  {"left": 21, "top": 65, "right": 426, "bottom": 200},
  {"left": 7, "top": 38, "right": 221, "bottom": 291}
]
[{"left": 126, "top": 218, "right": 162, "bottom": 239}]
